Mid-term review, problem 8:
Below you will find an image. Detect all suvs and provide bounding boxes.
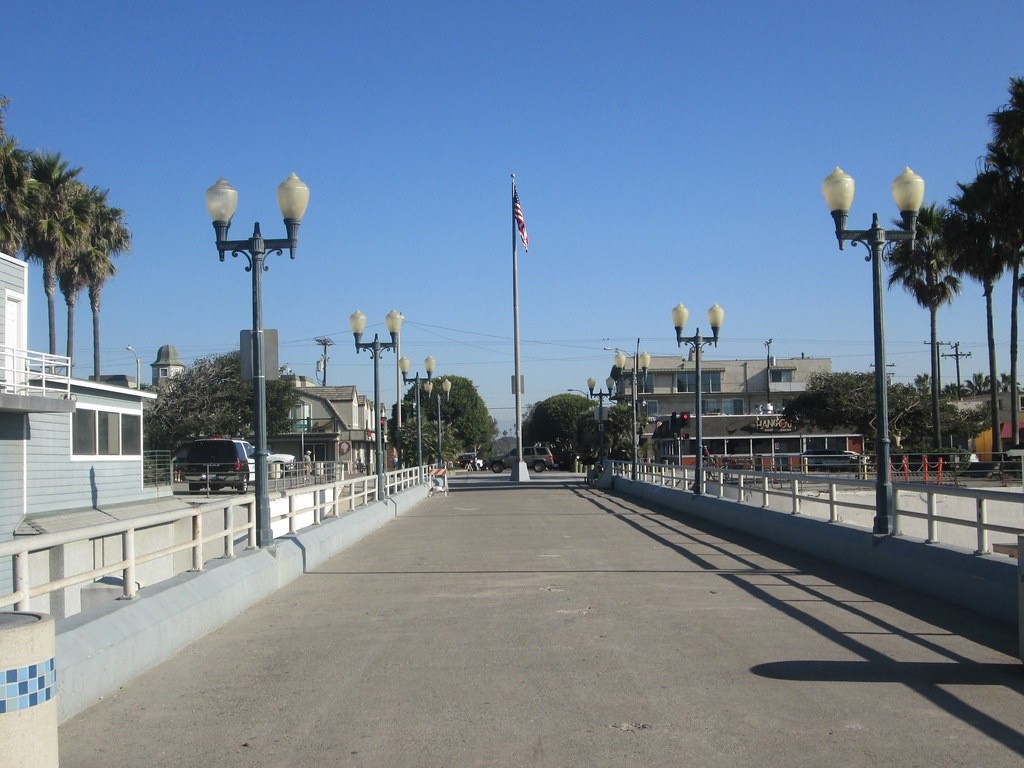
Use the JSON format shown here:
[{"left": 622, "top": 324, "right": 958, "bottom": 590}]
[
  {"left": 487, "top": 447, "right": 555, "bottom": 473},
  {"left": 252, "top": 445, "right": 296, "bottom": 479}
]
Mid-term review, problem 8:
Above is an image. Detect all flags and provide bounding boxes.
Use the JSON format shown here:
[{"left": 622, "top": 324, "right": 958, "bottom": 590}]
[{"left": 513, "top": 190, "right": 529, "bottom": 252}]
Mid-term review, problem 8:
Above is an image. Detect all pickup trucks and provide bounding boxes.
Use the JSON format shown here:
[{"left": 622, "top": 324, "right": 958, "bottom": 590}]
[{"left": 187, "top": 439, "right": 256, "bottom": 495}]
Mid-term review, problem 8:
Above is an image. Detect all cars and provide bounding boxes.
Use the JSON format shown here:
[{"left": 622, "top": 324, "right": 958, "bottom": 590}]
[
  {"left": 455, "top": 453, "right": 490, "bottom": 471},
  {"left": 801, "top": 449, "right": 875, "bottom": 472}
]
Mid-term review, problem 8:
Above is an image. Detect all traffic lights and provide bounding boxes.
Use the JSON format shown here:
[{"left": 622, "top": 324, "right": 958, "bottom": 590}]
[
  {"left": 381, "top": 417, "right": 386, "bottom": 432},
  {"left": 681, "top": 412, "right": 691, "bottom": 428}
]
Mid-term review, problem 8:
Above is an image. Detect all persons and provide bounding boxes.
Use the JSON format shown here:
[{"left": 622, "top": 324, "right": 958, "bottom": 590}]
[
  {"left": 722, "top": 455, "right": 737, "bottom": 470},
  {"left": 313, "top": 422, "right": 319, "bottom": 431},
  {"left": 304, "top": 451, "right": 312, "bottom": 482},
  {"left": 592, "top": 465, "right": 603, "bottom": 487},
  {"left": 704, "top": 446, "right": 712, "bottom": 479},
  {"left": 465, "top": 463, "right": 473, "bottom": 472},
  {"left": 393, "top": 455, "right": 398, "bottom": 469}
]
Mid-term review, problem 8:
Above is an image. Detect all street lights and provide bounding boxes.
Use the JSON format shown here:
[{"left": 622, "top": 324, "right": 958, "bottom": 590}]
[
  {"left": 587, "top": 377, "right": 615, "bottom": 468},
  {"left": 821, "top": 165, "right": 926, "bottom": 535},
  {"left": 425, "top": 378, "right": 452, "bottom": 463},
  {"left": 399, "top": 355, "right": 436, "bottom": 467},
  {"left": 126, "top": 346, "right": 140, "bottom": 391},
  {"left": 671, "top": 303, "right": 726, "bottom": 495},
  {"left": 350, "top": 309, "right": 405, "bottom": 501},
  {"left": 204, "top": 170, "right": 311, "bottom": 547},
  {"left": 566, "top": 388, "right": 589, "bottom": 411},
  {"left": 614, "top": 351, "right": 650, "bottom": 481}
]
